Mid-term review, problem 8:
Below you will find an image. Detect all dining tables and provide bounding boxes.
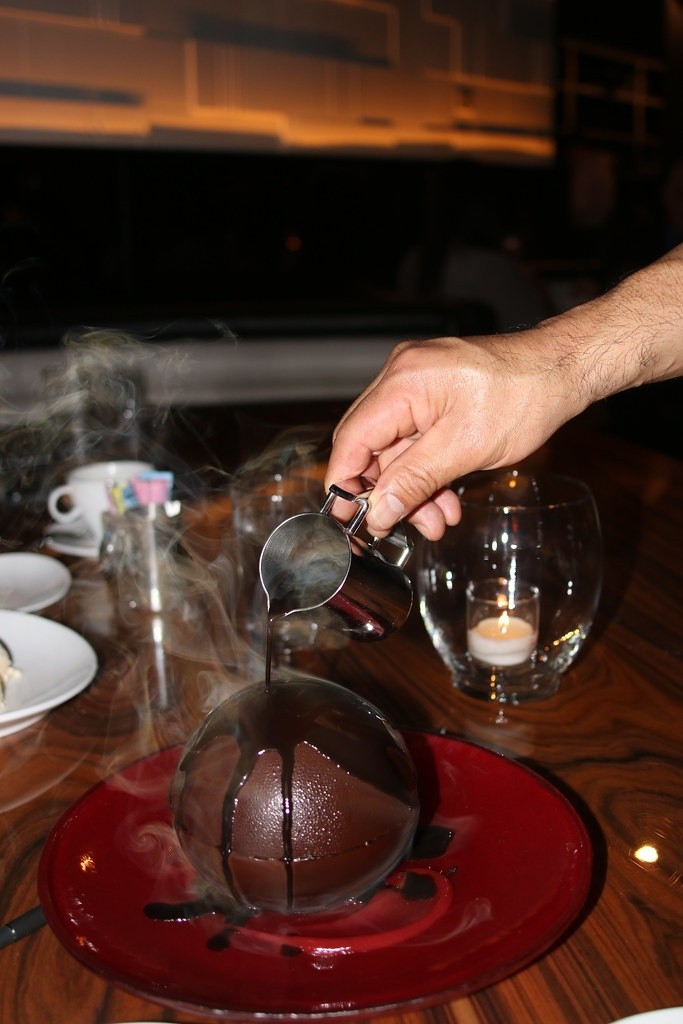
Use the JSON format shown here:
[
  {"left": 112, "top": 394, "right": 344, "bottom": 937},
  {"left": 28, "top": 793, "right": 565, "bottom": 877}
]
[{"left": 0, "top": 429, "right": 683, "bottom": 1024}]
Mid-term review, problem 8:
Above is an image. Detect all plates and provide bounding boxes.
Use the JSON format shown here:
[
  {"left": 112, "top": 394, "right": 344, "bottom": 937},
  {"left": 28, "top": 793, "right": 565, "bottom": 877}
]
[
  {"left": 37, "top": 534, "right": 100, "bottom": 559},
  {"left": 1, "top": 608, "right": 99, "bottom": 735},
  {"left": 37, "top": 719, "right": 592, "bottom": 1024},
  {"left": 0, "top": 554, "right": 73, "bottom": 615}
]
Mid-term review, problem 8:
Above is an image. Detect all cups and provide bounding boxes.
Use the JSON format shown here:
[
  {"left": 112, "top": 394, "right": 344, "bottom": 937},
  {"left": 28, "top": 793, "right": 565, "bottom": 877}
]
[
  {"left": 51, "top": 462, "right": 156, "bottom": 525},
  {"left": 423, "top": 475, "right": 609, "bottom": 706},
  {"left": 258, "top": 483, "right": 414, "bottom": 647},
  {"left": 235, "top": 472, "right": 315, "bottom": 546}
]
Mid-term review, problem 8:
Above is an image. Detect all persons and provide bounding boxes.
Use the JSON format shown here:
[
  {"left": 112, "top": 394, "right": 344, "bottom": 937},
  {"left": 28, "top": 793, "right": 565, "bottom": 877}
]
[{"left": 323, "top": 239, "right": 682, "bottom": 550}]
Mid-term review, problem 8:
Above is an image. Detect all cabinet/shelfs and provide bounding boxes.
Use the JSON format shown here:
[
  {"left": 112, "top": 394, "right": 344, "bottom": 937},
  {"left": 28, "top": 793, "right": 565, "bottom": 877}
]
[{"left": 560, "top": 37, "right": 674, "bottom": 153}]
